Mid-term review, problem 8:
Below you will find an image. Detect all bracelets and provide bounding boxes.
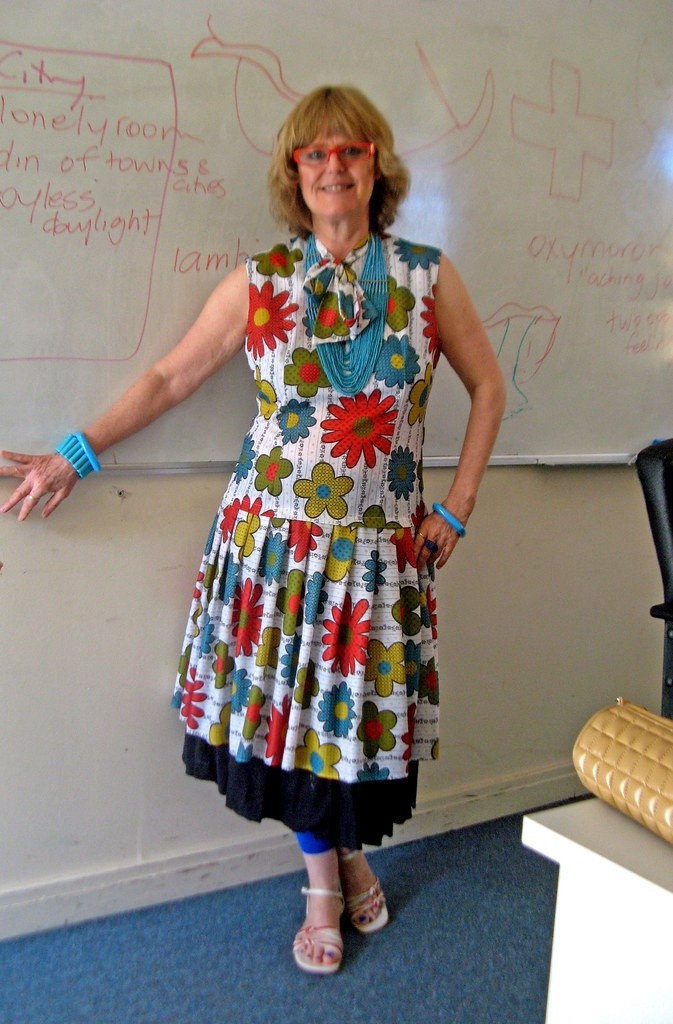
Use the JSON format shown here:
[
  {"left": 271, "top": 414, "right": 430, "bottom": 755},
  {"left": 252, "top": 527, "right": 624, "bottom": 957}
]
[
  {"left": 432, "top": 502, "right": 466, "bottom": 537},
  {"left": 55, "top": 431, "right": 101, "bottom": 479}
]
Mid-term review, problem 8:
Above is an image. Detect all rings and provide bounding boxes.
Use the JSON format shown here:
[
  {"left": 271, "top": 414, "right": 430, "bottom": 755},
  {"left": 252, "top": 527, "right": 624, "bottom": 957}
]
[
  {"left": 28, "top": 496, "right": 40, "bottom": 502},
  {"left": 417, "top": 532, "right": 426, "bottom": 539},
  {"left": 425, "top": 540, "right": 438, "bottom": 553}
]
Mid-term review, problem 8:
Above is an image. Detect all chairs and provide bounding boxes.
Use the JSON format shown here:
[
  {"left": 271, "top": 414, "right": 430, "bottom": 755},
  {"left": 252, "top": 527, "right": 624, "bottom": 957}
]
[{"left": 636, "top": 438, "right": 673, "bottom": 721}]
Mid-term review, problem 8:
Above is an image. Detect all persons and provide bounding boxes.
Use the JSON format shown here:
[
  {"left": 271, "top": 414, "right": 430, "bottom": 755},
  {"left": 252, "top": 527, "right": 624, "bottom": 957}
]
[{"left": 0, "top": 85, "right": 506, "bottom": 974}]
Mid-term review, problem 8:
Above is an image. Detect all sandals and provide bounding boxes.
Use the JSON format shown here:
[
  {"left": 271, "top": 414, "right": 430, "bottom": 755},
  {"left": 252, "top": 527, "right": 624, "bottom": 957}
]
[
  {"left": 338, "top": 848, "right": 389, "bottom": 934},
  {"left": 293, "top": 885, "right": 345, "bottom": 975}
]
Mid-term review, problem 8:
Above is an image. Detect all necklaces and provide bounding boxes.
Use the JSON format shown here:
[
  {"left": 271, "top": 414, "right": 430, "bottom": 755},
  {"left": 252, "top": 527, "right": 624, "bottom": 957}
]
[{"left": 304, "top": 233, "right": 388, "bottom": 396}]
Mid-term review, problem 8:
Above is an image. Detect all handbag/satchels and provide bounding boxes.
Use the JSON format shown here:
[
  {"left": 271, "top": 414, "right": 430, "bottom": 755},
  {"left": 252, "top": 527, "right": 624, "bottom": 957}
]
[{"left": 572, "top": 695, "right": 673, "bottom": 847}]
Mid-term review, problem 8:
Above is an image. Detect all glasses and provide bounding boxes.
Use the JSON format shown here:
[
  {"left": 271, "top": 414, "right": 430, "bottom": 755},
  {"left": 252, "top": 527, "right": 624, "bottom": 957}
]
[{"left": 293, "top": 142, "right": 377, "bottom": 168}]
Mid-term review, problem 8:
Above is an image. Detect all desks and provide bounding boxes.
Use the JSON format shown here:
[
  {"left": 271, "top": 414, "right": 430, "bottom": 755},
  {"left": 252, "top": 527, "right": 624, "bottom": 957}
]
[{"left": 521, "top": 796, "right": 673, "bottom": 1024}]
